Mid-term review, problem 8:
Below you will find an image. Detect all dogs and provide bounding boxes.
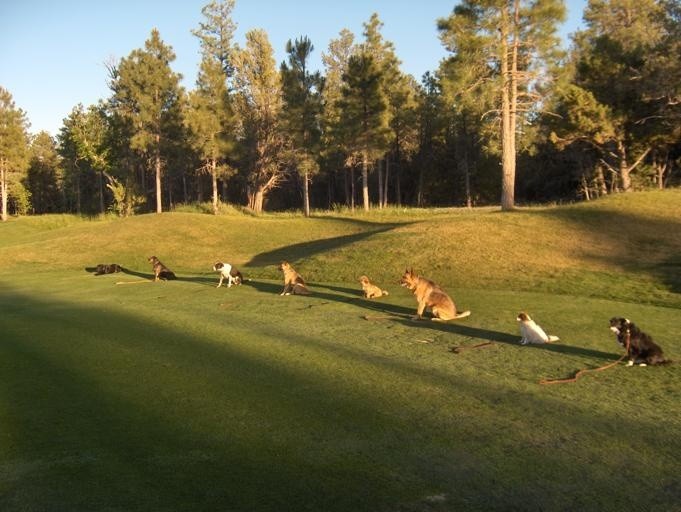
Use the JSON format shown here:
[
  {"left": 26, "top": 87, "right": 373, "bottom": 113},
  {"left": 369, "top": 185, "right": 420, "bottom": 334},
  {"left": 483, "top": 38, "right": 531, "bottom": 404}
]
[
  {"left": 398, "top": 267, "right": 472, "bottom": 322},
  {"left": 514, "top": 309, "right": 559, "bottom": 346},
  {"left": 609, "top": 316, "right": 674, "bottom": 368},
  {"left": 358, "top": 275, "right": 390, "bottom": 299},
  {"left": 148, "top": 255, "right": 176, "bottom": 282},
  {"left": 211, "top": 260, "right": 253, "bottom": 289},
  {"left": 277, "top": 259, "right": 315, "bottom": 296},
  {"left": 94, "top": 264, "right": 122, "bottom": 276}
]
